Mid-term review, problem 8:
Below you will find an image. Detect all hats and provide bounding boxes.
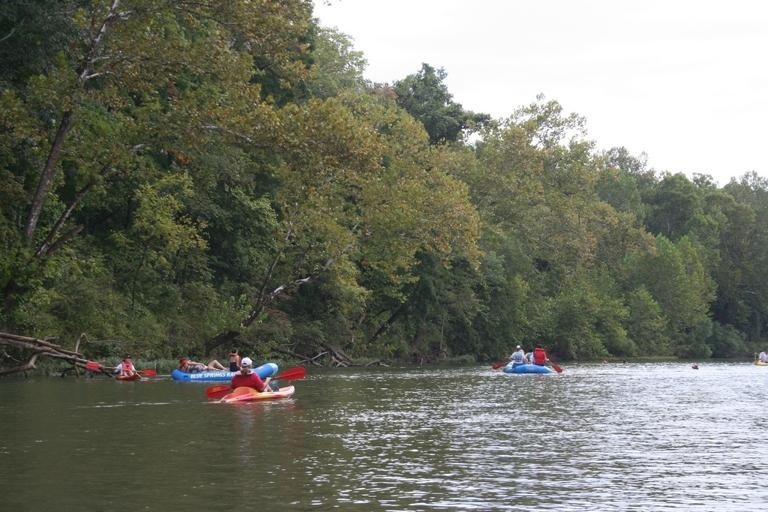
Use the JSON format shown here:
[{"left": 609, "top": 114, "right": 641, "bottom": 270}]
[
  {"left": 124, "top": 353, "right": 132, "bottom": 359},
  {"left": 242, "top": 358, "right": 252, "bottom": 366}
]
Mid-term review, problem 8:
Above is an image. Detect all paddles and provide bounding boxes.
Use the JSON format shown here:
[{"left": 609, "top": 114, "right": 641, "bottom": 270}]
[
  {"left": 492, "top": 363, "right": 508, "bottom": 369},
  {"left": 547, "top": 361, "right": 562, "bottom": 373},
  {"left": 206, "top": 368, "right": 304, "bottom": 398},
  {"left": 86, "top": 361, "right": 156, "bottom": 376}
]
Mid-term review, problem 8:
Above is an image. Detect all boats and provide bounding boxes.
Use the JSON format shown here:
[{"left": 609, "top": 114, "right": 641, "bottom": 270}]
[
  {"left": 220, "top": 386, "right": 296, "bottom": 403},
  {"left": 117, "top": 374, "right": 142, "bottom": 382},
  {"left": 172, "top": 361, "right": 281, "bottom": 383},
  {"left": 752, "top": 360, "right": 768, "bottom": 366},
  {"left": 504, "top": 360, "right": 555, "bottom": 374}
]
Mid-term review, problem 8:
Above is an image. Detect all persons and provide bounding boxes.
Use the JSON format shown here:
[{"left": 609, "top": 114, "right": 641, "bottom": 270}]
[
  {"left": 228, "top": 348, "right": 240, "bottom": 370},
  {"left": 759, "top": 350, "right": 768, "bottom": 363},
  {"left": 113, "top": 354, "right": 136, "bottom": 377},
  {"left": 231, "top": 357, "right": 270, "bottom": 390},
  {"left": 509, "top": 344, "right": 551, "bottom": 366},
  {"left": 179, "top": 359, "right": 226, "bottom": 370}
]
